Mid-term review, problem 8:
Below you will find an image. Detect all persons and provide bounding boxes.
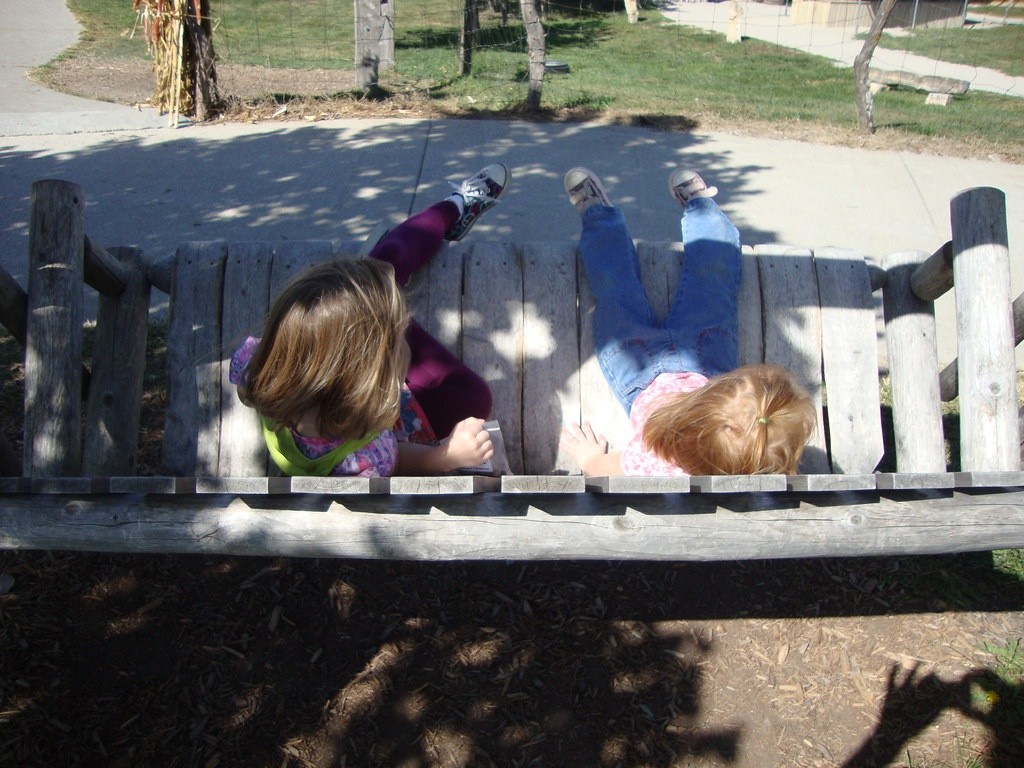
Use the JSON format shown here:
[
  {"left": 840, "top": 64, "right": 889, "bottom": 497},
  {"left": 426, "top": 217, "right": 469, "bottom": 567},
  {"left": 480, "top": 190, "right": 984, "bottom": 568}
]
[
  {"left": 554, "top": 165, "right": 818, "bottom": 476},
  {"left": 229, "top": 158, "right": 494, "bottom": 477}
]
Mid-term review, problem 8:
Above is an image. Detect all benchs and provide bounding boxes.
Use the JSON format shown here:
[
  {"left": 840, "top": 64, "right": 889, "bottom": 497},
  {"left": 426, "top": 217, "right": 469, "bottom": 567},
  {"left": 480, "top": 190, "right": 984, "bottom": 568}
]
[{"left": 0, "top": 177, "right": 1024, "bottom": 561}]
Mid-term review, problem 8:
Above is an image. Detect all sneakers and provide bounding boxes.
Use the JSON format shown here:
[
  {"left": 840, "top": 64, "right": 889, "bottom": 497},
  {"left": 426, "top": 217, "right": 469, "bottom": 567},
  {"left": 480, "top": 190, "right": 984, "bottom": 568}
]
[
  {"left": 442, "top": 161, "right": 513, "bottom": 243},
  {"left": 564, "top": 166, "right": 615, "bottom": 215},
  {"left": 668, "top": 167, "right": 719, "bottom": 212}
]
